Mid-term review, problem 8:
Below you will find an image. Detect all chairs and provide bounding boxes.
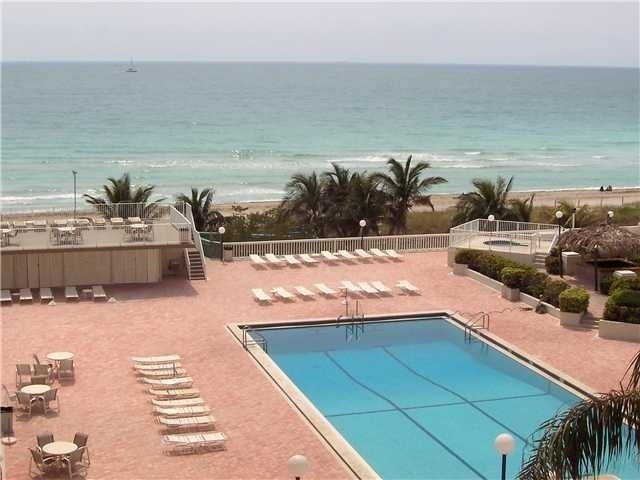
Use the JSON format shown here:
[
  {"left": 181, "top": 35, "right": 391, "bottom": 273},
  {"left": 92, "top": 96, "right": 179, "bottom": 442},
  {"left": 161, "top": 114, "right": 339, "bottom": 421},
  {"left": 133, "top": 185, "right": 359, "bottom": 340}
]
[
  {"left": 0, "top": 217, "right": 154, "bottom": 305},
  {"left": 0, "top": 352, "right": 91, "bottom": 480},
  {"left": 248, "top": 248, "right": 406, "bottom": 266},
  {"left": 251, "top": 279, "right": 422, "bottom": 304},
  {"left": 132, "top": 354, "right": 228, "bottom": 456}
]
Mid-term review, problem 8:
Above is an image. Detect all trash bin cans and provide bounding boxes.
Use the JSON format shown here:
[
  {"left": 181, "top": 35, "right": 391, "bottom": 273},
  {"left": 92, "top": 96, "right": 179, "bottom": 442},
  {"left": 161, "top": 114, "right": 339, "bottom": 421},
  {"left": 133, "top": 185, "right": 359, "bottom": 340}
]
[
  {"left": 0, "top": 406, "right": 14, "bottom": 437},
  {"left": 224, "top": 245, "right": 233, "bottom": 261}
]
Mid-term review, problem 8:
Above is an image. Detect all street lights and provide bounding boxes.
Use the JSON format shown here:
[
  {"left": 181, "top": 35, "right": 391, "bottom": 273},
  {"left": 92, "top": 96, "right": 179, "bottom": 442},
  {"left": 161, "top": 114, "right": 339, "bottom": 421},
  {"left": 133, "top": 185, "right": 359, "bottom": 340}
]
[
  {"left": 359, "top": 219, "right": 366, "bottom": 248},
  {"left": 287, "top": 455, "right": 309, "bottom": 480},
  {"left": 495, "top": 433, "right": 514, "bottom": 480},
  {"left": 607, "top": 211, "right": 614, "bottom": 224},
  {"left": 555, "top": 211, "right": 564, "bottom": 278},
  {"left": 488, "top": 214, "right": 494, "bottom": 231},
  {"left": 219, "top": 226, "right": 226, "bottom": 262}
]
